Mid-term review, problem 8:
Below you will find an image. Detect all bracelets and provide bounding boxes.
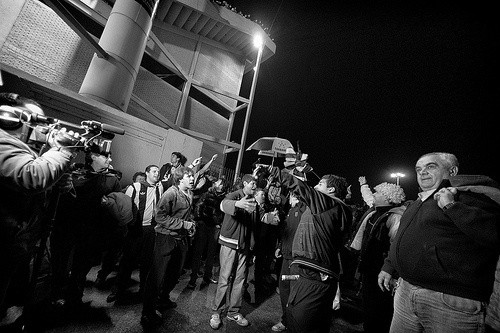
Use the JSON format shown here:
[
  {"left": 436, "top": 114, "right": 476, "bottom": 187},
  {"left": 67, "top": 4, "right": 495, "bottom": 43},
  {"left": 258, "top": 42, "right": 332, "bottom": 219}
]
[{"left": 440, "top": 202, "right": 459, "bottom": 215}]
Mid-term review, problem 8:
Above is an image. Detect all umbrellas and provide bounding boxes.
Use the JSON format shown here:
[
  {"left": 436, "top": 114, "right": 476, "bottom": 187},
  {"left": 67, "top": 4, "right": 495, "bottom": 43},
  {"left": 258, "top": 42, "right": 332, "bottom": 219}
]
[{"left": 246, "top": 133, "right": 296, "bottom": 178}]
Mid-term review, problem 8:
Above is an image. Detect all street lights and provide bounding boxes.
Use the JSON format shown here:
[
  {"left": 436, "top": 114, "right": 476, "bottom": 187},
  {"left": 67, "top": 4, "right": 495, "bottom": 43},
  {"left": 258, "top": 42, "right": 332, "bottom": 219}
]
[
  {"left": 390, "top": 172, "right": 406, "bottom": 186},
  {"left": 232, "top": 31, "right": 267, "bottom": 188}
]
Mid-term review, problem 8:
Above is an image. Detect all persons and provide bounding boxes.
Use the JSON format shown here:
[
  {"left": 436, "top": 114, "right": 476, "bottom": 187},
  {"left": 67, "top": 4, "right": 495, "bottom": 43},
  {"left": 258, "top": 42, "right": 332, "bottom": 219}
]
[
  {"left": 377, "top": 152, "right": 500, "bottom": 333},
  {"left": 188, "top": 153, "right": 219, "bottom": 193},
  {"left": 348, "top": 176, "right": 406, "bottom": 333},
  {"left": 121, "top": 171, "right": 146, "bottom": 195},
  {"left": 270, "top": 191, "right": 307, "bottom": 331},
  {"left": 255, "top": 164, "right": 353, "bottom": 333},
  {"left": 187, "top": 177, "right": 227, "bottom": 291},
  {"left": 0, "top": 94, "right": 87, "bottom": 333},
  {"left": 160, "top": 152, "right": 186, "bottom": 194},
  {"left": 42, "top": 144, "right": 133, "bottom": 333},
  {"left": 209, "top": 177, "right": 281, "bottom": 329},
  {"left": 107, "top": 165, "right": 161, "bottom": 304},
  {"left": 255, "top": 188, "right": 268, "bottom": 212},
  {"left": 140, "top": 168, "right": 197, "bottom": 326}
]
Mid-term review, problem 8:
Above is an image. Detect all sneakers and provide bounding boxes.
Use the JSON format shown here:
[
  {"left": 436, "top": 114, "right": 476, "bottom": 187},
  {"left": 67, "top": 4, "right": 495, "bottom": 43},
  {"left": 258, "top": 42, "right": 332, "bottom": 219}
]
[
  {"left": 227, "top": 313, "right": 248, "bottom": 326},
  {"left": 272, "top": 322, "right": 285, "bottom": 331},
  {"left": 210, "top": 314, "right": 220, "bottom": 328}
]
